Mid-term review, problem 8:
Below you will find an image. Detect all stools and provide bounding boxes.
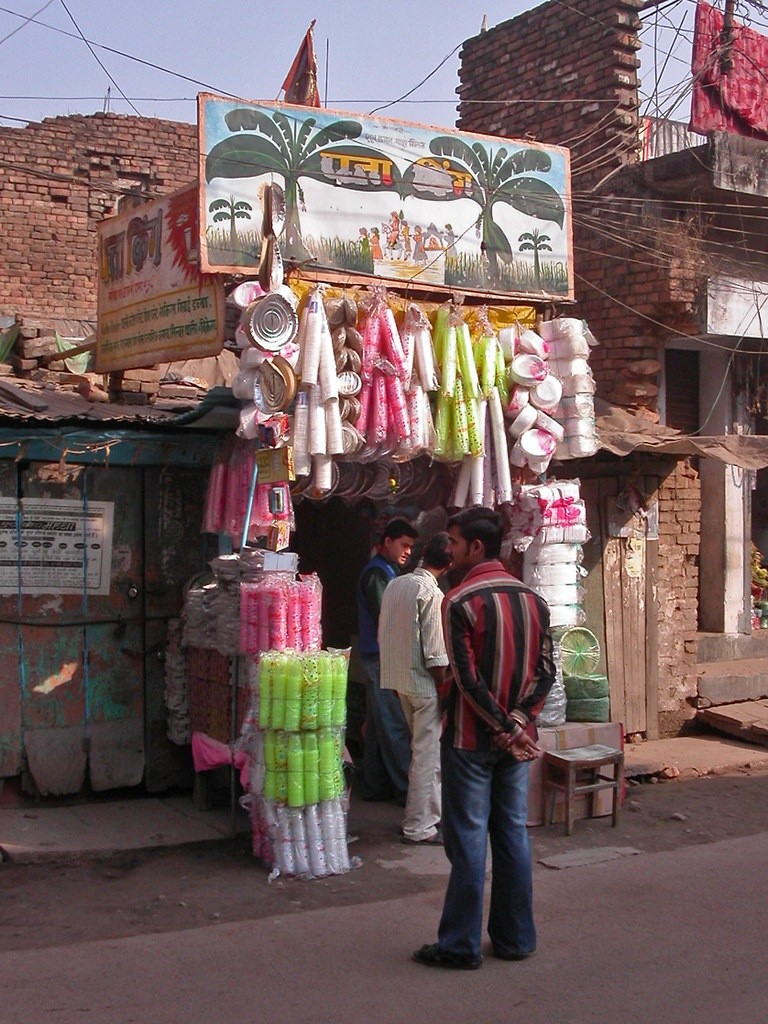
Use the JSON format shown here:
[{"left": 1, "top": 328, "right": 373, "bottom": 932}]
[{"left": 543, "top": 743, "right": 623, "bottom": 835}]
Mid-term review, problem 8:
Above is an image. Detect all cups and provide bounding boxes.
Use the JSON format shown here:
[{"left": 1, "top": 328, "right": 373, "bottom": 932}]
[
  {"left": 204, "top": 456, "right": 293, "bottom": 548},
  {"left": 238, "top": 572, "right": 352, "bottom": 883},
  {"left": 354, "top": 305, "right": 513, "bottom": 511},
  {"left": 291, "top": 291, "right": 343, "bottom": 491}
]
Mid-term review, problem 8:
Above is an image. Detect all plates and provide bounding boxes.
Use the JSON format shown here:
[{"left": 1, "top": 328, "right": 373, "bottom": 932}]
[{"left": 552, "top": 627, "right": 600, "bottom": 678}]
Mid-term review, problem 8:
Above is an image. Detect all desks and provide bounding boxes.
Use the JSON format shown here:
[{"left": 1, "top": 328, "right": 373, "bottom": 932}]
[{"left": 528, "top": 721, "right": 626, "bottom": 826}]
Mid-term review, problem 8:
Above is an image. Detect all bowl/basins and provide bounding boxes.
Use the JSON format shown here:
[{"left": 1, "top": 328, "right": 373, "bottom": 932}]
[
  {"left": 323, "top": 294, "right": 366, "bottom": 454},
  {"left": 498, "top": 324, "right": 565, "bottom": 472}
]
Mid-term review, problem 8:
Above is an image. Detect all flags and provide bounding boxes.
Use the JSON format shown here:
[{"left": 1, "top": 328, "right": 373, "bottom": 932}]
[{"left": 281, "top": 28, "right": 321, "bottom": 108}]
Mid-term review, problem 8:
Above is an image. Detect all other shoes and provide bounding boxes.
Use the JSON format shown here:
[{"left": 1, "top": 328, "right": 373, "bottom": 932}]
[
  {"left": 400, "top": 821, "right": 444, "bottom": 846},
  {"left": 413, "top": 943, "right": 483, "bottom": 970},
  {"left": 492, "top": 942, "right": 532, "bottom": 961},
  {"left": 363, "top": 789, "right": 407, "bottom": 807}
]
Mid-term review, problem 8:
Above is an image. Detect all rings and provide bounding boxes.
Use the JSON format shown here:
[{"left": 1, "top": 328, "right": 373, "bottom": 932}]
[{"left": 524, "top": 752, "right": 528, "bottom": 755}]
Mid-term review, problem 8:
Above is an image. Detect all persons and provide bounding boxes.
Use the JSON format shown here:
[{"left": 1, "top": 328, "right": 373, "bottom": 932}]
[
  {"left": 415, "top": 505, "right": 555, "bottom": 971},
  {"left": 355, "top": 518, "right": 421, "bottom": 805},
  {"left": 376, "top": 531, "right": 455, "bottom": 847}
]
